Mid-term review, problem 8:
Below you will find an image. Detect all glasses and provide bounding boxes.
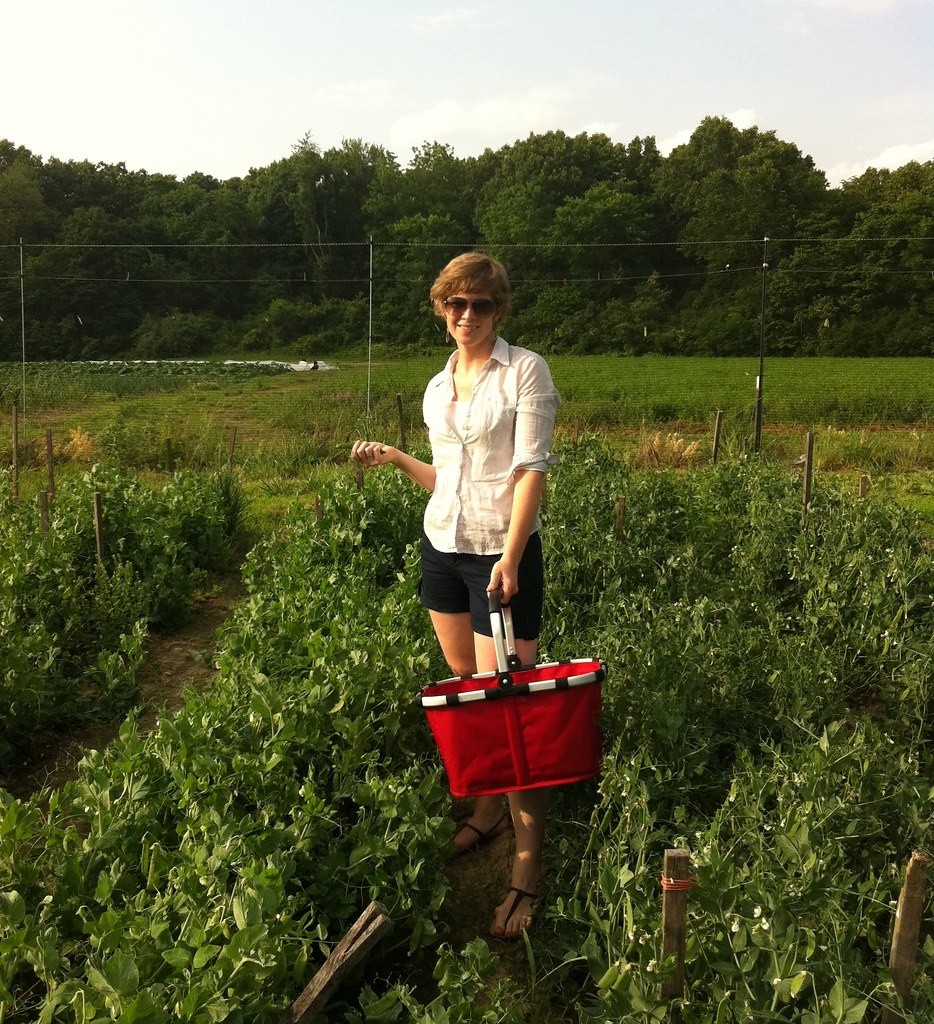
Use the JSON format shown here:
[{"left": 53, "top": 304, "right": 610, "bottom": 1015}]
[{"left": 444, "top": 296, "right": 500, "bottom": 319}]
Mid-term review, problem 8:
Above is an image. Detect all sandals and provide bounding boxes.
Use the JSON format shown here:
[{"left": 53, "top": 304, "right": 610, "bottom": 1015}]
[
  {"left": 488, "top": 886, "right": 538, "bottom": 938},
  {"left": 449, "top": 814, "right": 509, "bottom": 855}
]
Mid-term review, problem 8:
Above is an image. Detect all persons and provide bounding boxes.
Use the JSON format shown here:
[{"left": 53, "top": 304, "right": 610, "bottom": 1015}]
[{"left": 350, "top": 253, "right": 561, "bottom": 939}]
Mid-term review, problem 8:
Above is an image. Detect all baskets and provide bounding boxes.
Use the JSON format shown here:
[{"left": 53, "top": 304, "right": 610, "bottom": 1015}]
[{"left": 422, "top": 588, "right": 608, "bottom": 798}]
